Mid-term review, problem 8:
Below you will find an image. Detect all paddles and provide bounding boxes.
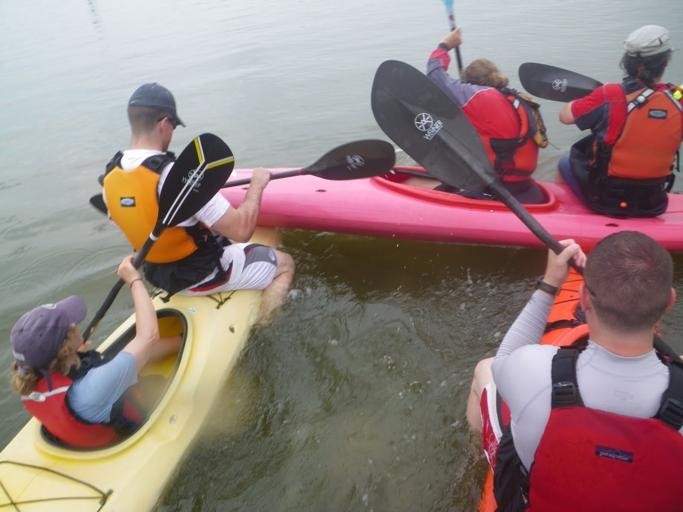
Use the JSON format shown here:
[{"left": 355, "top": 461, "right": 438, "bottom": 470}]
[
  {"left": 520, "top": 61, "right": 606, "bottom": 101},
  {"left": 370, "top": 60, "right": 682, "bottom": 369},
  {"left": 90, "top": 139, "right": 395, "bottom": 218},
  {"left": 81, "top": 132, "right": 234, "bottom": 344}
]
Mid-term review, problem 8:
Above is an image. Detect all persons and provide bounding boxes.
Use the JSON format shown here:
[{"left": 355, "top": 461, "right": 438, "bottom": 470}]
[
  {"left": 102, "top": 81, "right": 296, "bottom": 317},
  {"left": 466, "top": 231, "right": 683, "bottom": 512},
  {"left": 10, "top": 255, "right": 183, "bottom": 450},
  {"left": 557, "top": 25, "right": 683, "bottom": 218},
  {"left": 427, "top": 25, "right": 539, "bottom": 202}
]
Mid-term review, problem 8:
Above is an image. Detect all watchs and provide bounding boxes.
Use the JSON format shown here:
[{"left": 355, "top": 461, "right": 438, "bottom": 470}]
[{"left": 535, "top": 275, "right": 560, "bottom": 296}]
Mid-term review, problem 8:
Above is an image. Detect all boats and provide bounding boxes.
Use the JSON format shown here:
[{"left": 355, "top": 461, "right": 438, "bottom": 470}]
[
  {"left": 0, "top": 219, "right": 277, "bottom": 511},
  {"left": 223, "top": 163, "right": 682, "bottom": 253},
  {"left": 478, "top": 259, "right": 668, "bottom": 512}
]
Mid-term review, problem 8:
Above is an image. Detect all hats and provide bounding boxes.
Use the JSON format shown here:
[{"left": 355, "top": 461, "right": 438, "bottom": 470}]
[
  {"left": 624, "top": 25, "right": 675, "bottom": 57},
  {"left": 128, "top": 83, "right": 186, "bottom": 128},
  {"left": 10, "top": 295, "right": 86, "bottom": 369}
]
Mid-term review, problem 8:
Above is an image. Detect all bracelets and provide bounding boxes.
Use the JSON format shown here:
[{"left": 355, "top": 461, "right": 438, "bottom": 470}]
[{"left": 130, "top": 278, "right": 143, "bottom": 288}]
